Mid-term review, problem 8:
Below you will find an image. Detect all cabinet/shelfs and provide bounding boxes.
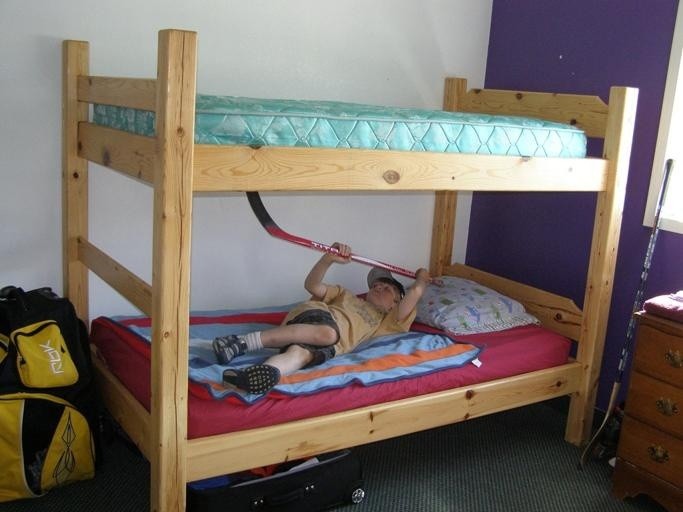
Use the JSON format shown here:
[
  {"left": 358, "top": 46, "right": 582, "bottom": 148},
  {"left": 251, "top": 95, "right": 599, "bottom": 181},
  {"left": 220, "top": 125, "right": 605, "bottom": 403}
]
[{"left": 611, "top": 311, "right": 683, "bottom": 511}]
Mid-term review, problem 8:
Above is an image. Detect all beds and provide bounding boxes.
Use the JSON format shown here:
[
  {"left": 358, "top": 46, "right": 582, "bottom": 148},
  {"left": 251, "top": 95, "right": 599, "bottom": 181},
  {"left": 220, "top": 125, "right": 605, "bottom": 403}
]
[{"left": 61, "top": 29, "right": 641, "bottom": 510}]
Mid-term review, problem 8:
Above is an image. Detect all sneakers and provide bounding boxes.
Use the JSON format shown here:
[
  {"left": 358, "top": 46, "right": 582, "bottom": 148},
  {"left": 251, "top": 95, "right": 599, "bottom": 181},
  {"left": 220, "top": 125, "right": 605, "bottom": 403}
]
[
  {"left": 223, "top": 364, "right": 281, "bottom": 394},
  {"left": 213, "top": 334, "right": 247, "bottom": 365}
]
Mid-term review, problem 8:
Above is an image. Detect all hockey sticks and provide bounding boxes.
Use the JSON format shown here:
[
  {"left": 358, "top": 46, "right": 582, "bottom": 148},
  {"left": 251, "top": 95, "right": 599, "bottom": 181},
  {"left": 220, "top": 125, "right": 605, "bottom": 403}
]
[
  {"left": 580, "top": 158, "right": 673, "bottom": 469},
  {"left": 246, "top": 192, "right": 444, "bottom": 288}
]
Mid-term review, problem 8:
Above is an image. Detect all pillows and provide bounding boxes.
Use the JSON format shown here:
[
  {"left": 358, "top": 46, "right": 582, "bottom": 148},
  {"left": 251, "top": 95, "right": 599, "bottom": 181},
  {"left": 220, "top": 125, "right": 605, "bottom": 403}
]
[{"left": 401, "top": 276, "right": 539, "bottom": 336}]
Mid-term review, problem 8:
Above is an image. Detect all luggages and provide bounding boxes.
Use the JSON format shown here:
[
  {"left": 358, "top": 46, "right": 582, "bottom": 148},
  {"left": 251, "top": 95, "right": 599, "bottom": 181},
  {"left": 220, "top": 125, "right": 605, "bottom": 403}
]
[{"left": 186, "top": 448, "right": 365, "bottom": 512}]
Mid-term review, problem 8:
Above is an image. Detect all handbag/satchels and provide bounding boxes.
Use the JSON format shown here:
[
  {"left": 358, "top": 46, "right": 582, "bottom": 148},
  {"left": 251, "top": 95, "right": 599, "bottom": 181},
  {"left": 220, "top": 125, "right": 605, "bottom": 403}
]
[{"left": 3, "top": 395, "right": 97, "bottom": 500}]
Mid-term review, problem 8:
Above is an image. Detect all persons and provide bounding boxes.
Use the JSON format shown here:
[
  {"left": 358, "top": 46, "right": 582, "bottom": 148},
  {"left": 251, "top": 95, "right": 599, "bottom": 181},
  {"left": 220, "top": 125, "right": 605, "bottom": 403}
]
[{"left": 211, "top": 240, "right": 431, "bottom": 396}]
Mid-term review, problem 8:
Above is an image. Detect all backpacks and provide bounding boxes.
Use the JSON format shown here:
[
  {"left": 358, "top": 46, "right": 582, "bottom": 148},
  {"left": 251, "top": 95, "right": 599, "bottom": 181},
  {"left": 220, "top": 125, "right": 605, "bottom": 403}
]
[{"left": 0, "top": 286, "right": 101, "bottom": 394}]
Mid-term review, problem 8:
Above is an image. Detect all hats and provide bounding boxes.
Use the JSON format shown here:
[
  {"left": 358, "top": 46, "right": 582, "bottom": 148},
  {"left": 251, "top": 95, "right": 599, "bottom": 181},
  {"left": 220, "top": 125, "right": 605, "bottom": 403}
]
[{"left": 367, "top": 266, "right": 404, "bottom": 299}]
